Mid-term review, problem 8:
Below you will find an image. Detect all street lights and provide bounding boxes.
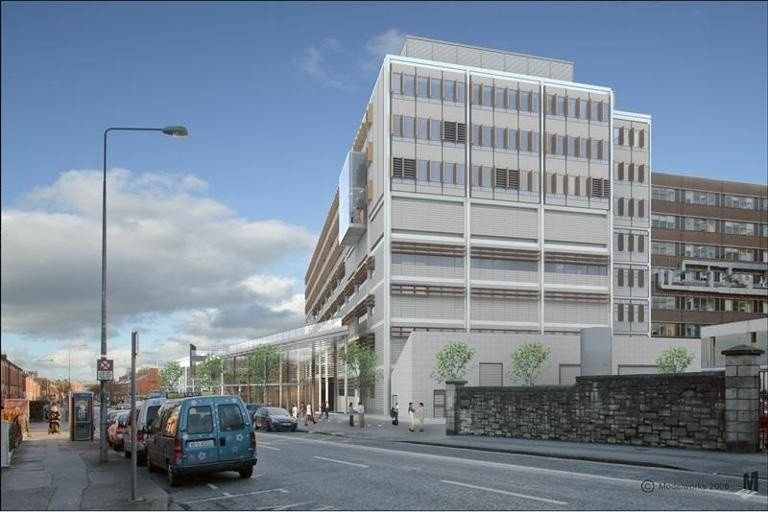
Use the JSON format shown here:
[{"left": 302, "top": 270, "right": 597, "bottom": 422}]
[
  {"left": 68, "top": 342, "right": 90, "bottom": 393},
  {"left": 98, "top": 122, "right": 192, "bottom": 469},
  {"left": 33, "top": 358, "right": 55, "bottom": 372}
]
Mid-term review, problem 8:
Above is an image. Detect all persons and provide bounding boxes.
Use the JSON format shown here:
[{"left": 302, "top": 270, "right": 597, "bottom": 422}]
[
  {"left": 417, "top": 401, "right": 425, "bottom": 431},
  {"left": 291, "top": 399, "right": 330, "bottom": 425},
  {"left": 48, "top": 404, "right": 62, "bottom": 435},
  {"left": 357, "top": 401, "right": 365, "bottom": 428},
  {"left": 349, "top": 402, "right": 355, "bottom": 426},
  {"left": 408, "top": 402, "right": 416, "bottom": 431},
  {"left": 394, "top": 401, "right": 399, "bottom": 421}
]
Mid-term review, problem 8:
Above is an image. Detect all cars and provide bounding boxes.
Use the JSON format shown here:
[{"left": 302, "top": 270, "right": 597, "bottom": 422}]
[{"left": 92, "top": 395, "right": 300, "bottom": 487}]
